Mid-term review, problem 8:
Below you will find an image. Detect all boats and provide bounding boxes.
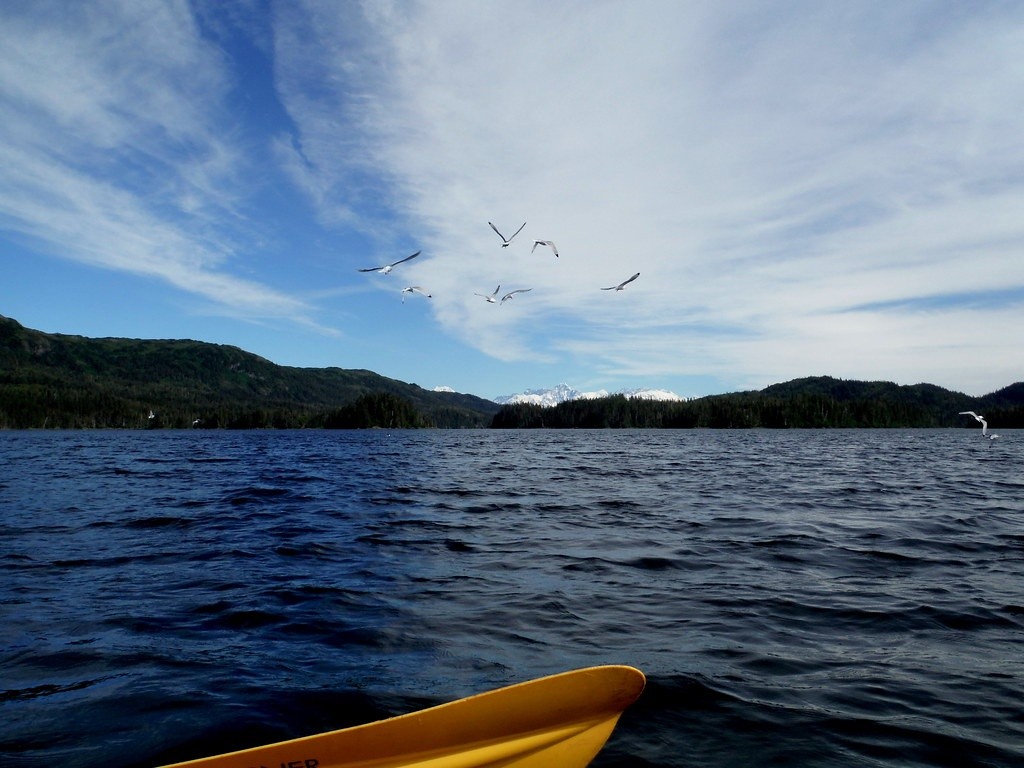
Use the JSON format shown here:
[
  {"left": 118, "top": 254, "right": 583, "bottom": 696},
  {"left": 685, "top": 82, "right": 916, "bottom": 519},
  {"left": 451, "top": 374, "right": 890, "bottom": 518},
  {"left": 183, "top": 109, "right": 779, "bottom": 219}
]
[{"left": 157, "top": 663, "right": 647, "bottom": 768}]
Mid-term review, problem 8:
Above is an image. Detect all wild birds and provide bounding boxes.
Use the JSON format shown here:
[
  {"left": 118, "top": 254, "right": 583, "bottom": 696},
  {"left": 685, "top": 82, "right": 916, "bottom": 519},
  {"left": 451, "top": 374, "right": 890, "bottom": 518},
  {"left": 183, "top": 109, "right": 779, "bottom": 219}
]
[
  {"left": 402, "top": 286, "right": 433, "bottom": 304},
  {"left": 473, "top": 283, "right": 535, "bottom": 310},
  {"left": 488, "top": 221, "right": 527, "bottom": 247},
  {"left": 530, "top": 240, "right": 559, "bottom": 257},
  {"left": 959, "top": 412, "right": 1002, "bottom": 440},
  {"left": 148, "top": 410, "right": 154, "bottom": 419},
  {"left": 600, "top": 273, "right": 640, "bottom": 291},
  {"left": 193, "top": 419, "right": 200, "bottom": 425},
  {"left": 357, "top": 250, "right": 422, "bottom": 274}
]
